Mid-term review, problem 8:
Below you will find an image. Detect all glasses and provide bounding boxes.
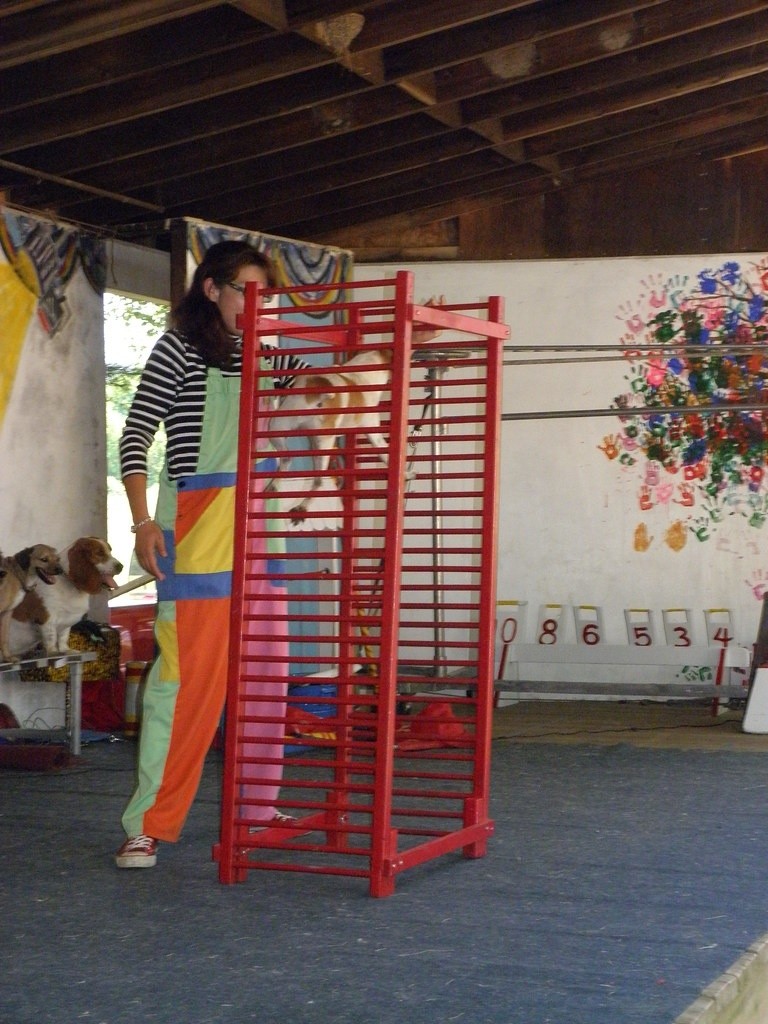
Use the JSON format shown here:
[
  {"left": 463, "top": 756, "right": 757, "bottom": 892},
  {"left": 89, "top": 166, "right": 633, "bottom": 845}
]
[{"left": 227, "top": 281, "right": 273, "bottom": 304}]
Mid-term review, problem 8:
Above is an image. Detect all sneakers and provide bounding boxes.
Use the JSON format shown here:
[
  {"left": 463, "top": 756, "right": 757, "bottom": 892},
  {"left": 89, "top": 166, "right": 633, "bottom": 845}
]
[
  {"left": 116, "top": 835, "right": 159, "bottom": 868},
  {"left": 248, "top": 812, "right": 313, "bottom": 837}
]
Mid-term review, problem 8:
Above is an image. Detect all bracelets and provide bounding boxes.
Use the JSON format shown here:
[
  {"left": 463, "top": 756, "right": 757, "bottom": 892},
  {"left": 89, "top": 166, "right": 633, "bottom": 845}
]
[{"left": 130, "top": 516, "right": 153, "bottom": 533}]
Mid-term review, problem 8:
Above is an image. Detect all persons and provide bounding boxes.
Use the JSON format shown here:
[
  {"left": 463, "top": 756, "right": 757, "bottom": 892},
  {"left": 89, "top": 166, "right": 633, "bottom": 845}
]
[{"left": 112, "top": 239, "right": 446, "bottom": 868}]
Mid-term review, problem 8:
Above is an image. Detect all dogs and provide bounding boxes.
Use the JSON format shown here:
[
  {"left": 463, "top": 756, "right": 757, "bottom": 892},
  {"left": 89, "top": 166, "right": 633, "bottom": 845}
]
[
  {"left": 1, "top": 544, "right": 65, "bottom": 662},
  {"left": 260, "top": 340, "right": 416, "bottom": 520},
  {"left": 1, "top": 536, "right": 124, "bottom": 656}
]
